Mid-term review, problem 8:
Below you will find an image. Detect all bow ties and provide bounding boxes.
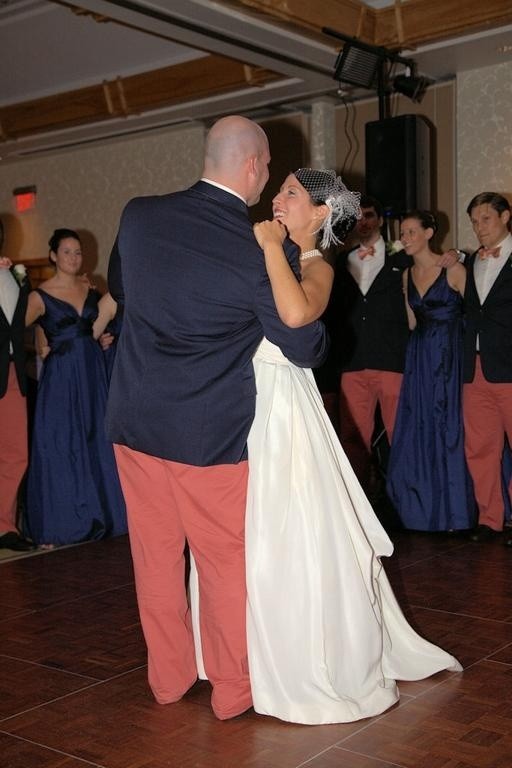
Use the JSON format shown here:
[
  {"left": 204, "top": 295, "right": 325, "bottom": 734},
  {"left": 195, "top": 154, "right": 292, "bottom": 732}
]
[
  {"left": 480, "top": 247, "right": 503, "bottom": 260},
  {"left": 356, "top": 246, "right": 376, "bottom": 260}
]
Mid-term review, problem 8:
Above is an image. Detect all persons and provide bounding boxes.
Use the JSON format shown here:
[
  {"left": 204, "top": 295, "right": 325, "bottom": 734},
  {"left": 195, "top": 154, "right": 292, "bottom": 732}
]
[
  {"left": 23, "top": 226, "right": 108, "bottom": 554},
  {"left": 326, "top": 193, "right": 473, "bottom": 498},
  {"left": 383, "top": 207, "right": 480, "bottom": 538},
  {"left": 99, "top": 329, "right": 116, "bottom": 353},
  {"left": 399, "top": 186, "right": 512, "bottom": 547},
  {"left": 87, "top": 282, "right": 132, "bottom": 544},
  {"left": 0, "top": 256, "right": 35, "bottom": 551},
  {"left": 103, "top": 110, "right": 341, "bottom": 724},
  {"left": 187, "top": 163, "right": 468, "bottom": 724}
]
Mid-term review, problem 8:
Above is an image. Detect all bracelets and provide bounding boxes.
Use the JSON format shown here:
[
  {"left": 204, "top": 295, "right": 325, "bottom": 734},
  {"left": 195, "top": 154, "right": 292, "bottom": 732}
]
[{"left": 455, "top": 247, "right": 461, "bottom": 258}]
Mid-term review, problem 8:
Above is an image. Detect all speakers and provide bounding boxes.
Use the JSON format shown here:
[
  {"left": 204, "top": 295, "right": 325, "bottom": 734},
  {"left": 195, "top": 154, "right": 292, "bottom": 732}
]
[
  {"left": 364, "top": 112, "right": 432, "bottom": 215},
  {"left": 259, "top": 121, "right": 303, "bottom": 200}
]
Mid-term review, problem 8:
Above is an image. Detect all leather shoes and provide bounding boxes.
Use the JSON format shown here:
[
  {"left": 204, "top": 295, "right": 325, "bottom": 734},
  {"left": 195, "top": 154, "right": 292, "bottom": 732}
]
[
  {"left": 0, "top": 531, "right": 39, "bottom": 552},
  {"left": 468, "top": 525, "right": 493, "bottom": 545}
]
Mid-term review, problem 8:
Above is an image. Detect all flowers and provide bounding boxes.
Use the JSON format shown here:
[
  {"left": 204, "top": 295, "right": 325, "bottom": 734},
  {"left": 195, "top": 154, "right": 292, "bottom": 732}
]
[
  {"left": 386, "top": 238, "right": 405, "bottom": 256},
  {"left": 13, "top": 264, "right": 28, "bottom": 287}
]
